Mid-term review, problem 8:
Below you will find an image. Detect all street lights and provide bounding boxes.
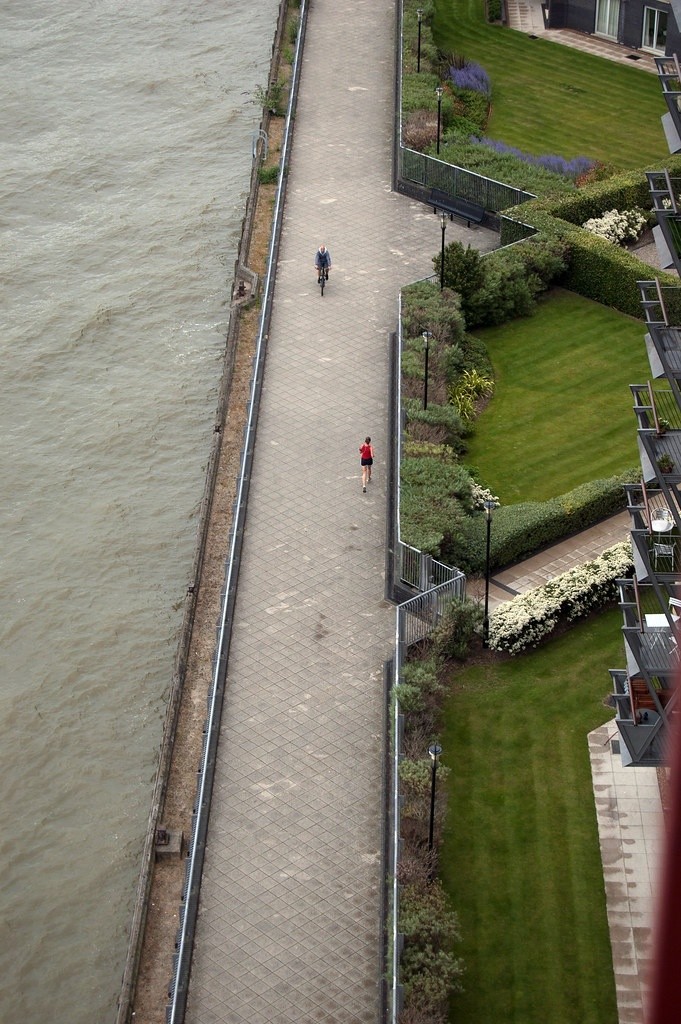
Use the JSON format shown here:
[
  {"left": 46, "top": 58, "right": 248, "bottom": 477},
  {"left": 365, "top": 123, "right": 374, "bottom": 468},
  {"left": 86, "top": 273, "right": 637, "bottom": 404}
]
[
  {"left": 435, "top": 88, "right": 446, "bottom": 155},
  {"left": 423, "top": 329, "right": 434, "bottom": 410},
  {"left": 483, "top": 497, "right": 495, "bottom": 649},
  {"left": 427, "top": 743, "right": 443, "bottom": 852},
  {"left": 416, "top": 8, "right": 424, "bottom": 73},
  {"left": 438, "top": 211, "right": 452, "bottom": 294}
]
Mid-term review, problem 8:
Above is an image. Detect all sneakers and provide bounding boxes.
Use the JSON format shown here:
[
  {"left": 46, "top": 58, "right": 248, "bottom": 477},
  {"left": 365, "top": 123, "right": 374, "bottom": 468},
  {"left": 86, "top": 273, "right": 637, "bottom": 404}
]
[
  {"left": 318, "top": 278, "right": 320, "bottom": 283},
  {"left": 363, "top": 486, "right": 366, "bottom": 493},
  {"left": 326, "top": 274, "right": 328, "bottom": 281},
  {"left": 367, "top": 477, "right": 372, "bottom": 483}
]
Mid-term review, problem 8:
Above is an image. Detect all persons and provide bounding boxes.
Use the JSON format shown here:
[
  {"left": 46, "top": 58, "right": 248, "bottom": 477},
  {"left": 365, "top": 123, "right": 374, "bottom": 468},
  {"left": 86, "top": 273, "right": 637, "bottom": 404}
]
[
  {"left": 315, "top": 245, "right": 332, "bottom": 283},
  {"left": 359, "top": 436, "right": 375, "bottom": 492}
]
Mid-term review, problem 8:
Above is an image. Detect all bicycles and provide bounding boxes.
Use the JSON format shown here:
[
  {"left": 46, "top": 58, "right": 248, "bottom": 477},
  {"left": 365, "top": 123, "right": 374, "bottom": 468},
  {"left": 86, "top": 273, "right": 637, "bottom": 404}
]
[{"left": 316, "top": 264, "right": 328, "bottom": 296}]
